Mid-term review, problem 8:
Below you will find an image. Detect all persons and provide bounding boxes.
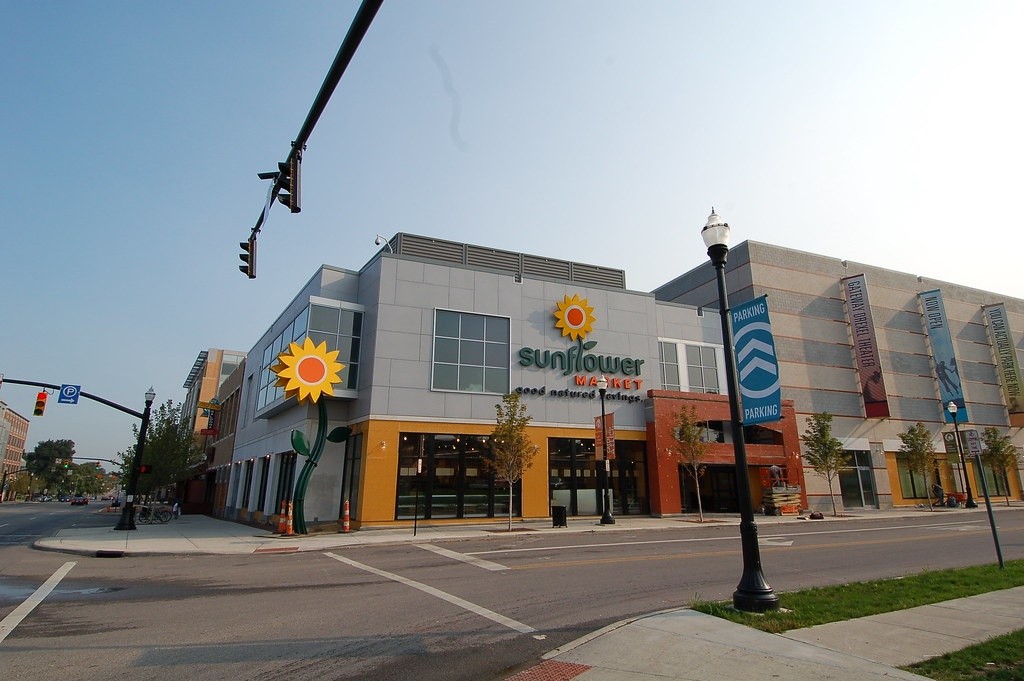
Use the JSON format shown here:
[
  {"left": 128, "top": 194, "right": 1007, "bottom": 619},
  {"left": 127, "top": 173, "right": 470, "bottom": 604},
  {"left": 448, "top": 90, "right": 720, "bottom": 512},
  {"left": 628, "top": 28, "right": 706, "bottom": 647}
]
[{"left": 173, "top": 497, "right": 183, "bottom": 518}]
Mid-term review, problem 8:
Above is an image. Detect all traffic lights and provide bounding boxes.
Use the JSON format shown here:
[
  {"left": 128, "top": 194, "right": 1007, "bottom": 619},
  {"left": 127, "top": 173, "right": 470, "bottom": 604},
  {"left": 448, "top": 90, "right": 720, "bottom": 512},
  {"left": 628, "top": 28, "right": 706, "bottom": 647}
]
[
  {"left": 64, "top": 460, "right": 68, "bottom": 469},
  {"left": 33, "top": 391, "right": 47, "bottom": 416},
  {"left": 239, "top": 149, "right": 302, "bottom": 279},
  {"left": 95, "top": 462, "right": 99, "bottom": 470},
  {"left": 141, "top": 465, "right": 152, "bottom": 474}
]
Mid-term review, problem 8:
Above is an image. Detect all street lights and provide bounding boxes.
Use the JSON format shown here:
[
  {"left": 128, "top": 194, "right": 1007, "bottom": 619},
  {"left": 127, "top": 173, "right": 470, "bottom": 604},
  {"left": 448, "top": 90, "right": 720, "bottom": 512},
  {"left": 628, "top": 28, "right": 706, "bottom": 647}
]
[
  {"left": 948, "top": 401, "right": 978, "bottom": 509},
  {"left": 597, "top": 374, "right": 615, "bottom": 524},
  {"left": 28, "top": 471, "right": 34, "bottom": 502},
  {"left": 114, "top": 386, "right": 156, "bottom": 530},
  {"left": 701, "top": 206, "right": 780, "bottom": 613}
]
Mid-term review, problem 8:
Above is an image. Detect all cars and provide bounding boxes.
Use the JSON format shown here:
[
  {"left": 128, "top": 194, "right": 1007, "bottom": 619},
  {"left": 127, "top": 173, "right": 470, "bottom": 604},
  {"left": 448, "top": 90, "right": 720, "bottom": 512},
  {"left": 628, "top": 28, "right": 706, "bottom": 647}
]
[
  {"left": 112, "top": 499, "right": 121, "bottom": 506},
  {"left": 71, "top": 495, "right": 88, "bottom": 505},
  {"left": 32, "top": 494, "right": 52, "bottom": 501},
  {"left": 59, "top": 496, "right": 70, "bottom": 502},
  {"left": 90, "top": 495, "right": 96, "bottom": 500},
  {"left": 108, "top": 495, "right": 113, "bottom": 500}
]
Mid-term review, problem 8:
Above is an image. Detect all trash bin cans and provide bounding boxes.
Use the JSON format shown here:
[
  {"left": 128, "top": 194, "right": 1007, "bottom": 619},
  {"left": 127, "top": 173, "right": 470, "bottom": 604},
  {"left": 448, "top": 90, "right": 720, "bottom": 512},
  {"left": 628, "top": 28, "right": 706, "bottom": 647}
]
[{"left": 551, "top": 506, "right": 568, "bottom": 528}]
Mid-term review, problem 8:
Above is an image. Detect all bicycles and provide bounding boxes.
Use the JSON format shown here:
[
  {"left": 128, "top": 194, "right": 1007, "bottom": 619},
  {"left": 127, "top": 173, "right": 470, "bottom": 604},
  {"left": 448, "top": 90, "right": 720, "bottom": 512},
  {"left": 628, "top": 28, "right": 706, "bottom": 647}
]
[{"left": 135, "top": 500, "right": 172, "bottom": 524}]
